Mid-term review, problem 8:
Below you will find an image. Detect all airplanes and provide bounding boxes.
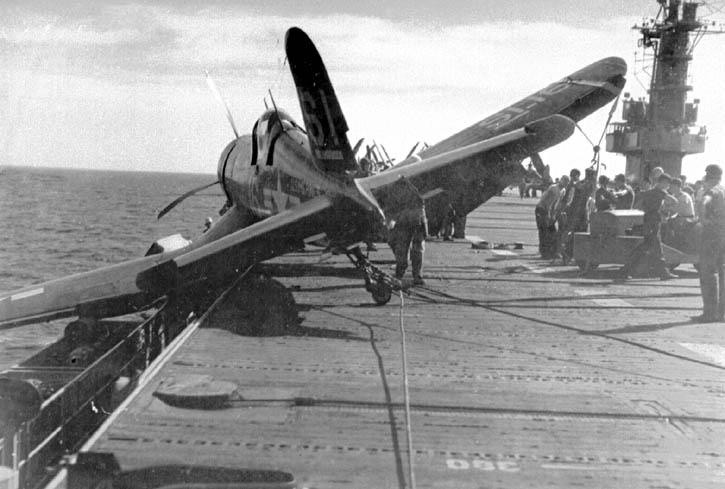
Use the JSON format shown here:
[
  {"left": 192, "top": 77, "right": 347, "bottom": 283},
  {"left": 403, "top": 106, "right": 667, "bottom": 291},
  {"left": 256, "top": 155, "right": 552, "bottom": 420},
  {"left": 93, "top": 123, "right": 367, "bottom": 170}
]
[
  {"left": 520, "top": 153, "right": 555, "bottom": 197},
  {"left": 0, "top": 25, "right": 628, "bottom": 338}
]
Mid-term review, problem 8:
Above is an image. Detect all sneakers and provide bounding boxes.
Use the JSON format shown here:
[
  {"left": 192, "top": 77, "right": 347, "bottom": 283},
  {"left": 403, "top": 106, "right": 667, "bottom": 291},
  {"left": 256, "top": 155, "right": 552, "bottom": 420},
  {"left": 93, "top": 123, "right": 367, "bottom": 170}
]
[
  {"left": 395, "top": 263, "right": 408, "bottom": 280},
  {"left": 690, "top": 310, "right": 725, "bottom": 323},
  {"left": 413, "top": 275, "right": 425, "bottom": 285},
  {"left": 431, "top": 231, "right": 465, "bottom": 243}
]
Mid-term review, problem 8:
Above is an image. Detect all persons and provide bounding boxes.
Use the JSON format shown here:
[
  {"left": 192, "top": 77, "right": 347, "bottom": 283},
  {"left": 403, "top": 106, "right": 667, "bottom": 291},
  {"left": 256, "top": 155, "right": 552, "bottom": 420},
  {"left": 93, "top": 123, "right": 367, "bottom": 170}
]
[
  {"left": 443, "top": 158, "right": 469, "bottom": 241},
  {"left": 690, "top": 165, "right": 724, "bottom": 321},
  {"left": 522, "top": 162, "right": 725, "bottom": 281},
  {"left": 357, "top": 155, "right": 428, "bottom": 283}
]
